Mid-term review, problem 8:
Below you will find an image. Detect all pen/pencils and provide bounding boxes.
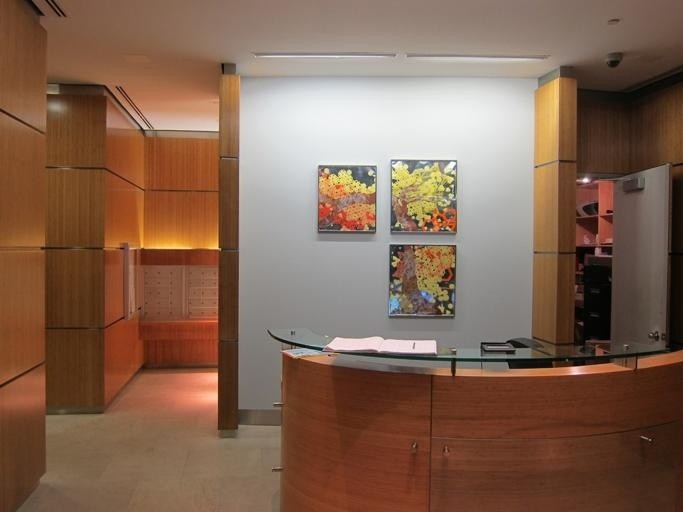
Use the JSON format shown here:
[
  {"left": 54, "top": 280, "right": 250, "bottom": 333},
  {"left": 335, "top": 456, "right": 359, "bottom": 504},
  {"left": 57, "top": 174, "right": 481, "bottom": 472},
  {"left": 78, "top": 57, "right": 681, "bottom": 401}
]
[{"left": 413, "top": 343, "right": 415, "bottom": 349}]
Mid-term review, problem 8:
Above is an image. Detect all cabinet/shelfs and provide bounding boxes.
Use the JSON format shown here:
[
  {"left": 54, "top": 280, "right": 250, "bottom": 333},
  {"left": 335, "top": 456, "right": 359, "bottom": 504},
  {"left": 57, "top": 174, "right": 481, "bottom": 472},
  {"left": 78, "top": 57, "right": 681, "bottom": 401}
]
[{"left": 575, "top": 182, "right": 613, "bottom": 342}]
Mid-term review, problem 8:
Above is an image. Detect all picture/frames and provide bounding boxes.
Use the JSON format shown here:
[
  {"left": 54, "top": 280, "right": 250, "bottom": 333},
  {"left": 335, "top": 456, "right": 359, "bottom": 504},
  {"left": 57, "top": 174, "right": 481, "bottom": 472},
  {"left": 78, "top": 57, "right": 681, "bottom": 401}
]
[
  {"left": 388, "top": 159, "right": 458, "bottom": 318},
  {"left": 318, "top": 164, "right": 378, "bottom": 234}
]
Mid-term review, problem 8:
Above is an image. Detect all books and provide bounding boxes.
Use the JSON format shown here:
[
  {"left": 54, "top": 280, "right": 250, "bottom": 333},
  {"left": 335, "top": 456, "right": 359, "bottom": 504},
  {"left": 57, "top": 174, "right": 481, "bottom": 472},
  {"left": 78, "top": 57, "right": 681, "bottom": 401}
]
[{"left": 322, "top": 336, "right": 437, "bottom": 357}]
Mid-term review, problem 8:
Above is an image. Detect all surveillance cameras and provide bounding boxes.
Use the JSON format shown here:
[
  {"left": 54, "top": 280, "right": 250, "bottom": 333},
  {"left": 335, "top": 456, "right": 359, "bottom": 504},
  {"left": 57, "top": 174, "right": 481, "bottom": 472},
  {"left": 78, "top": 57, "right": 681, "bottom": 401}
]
[{"left": 606, "top": 53, "right": 621, "bottom": 68}]
